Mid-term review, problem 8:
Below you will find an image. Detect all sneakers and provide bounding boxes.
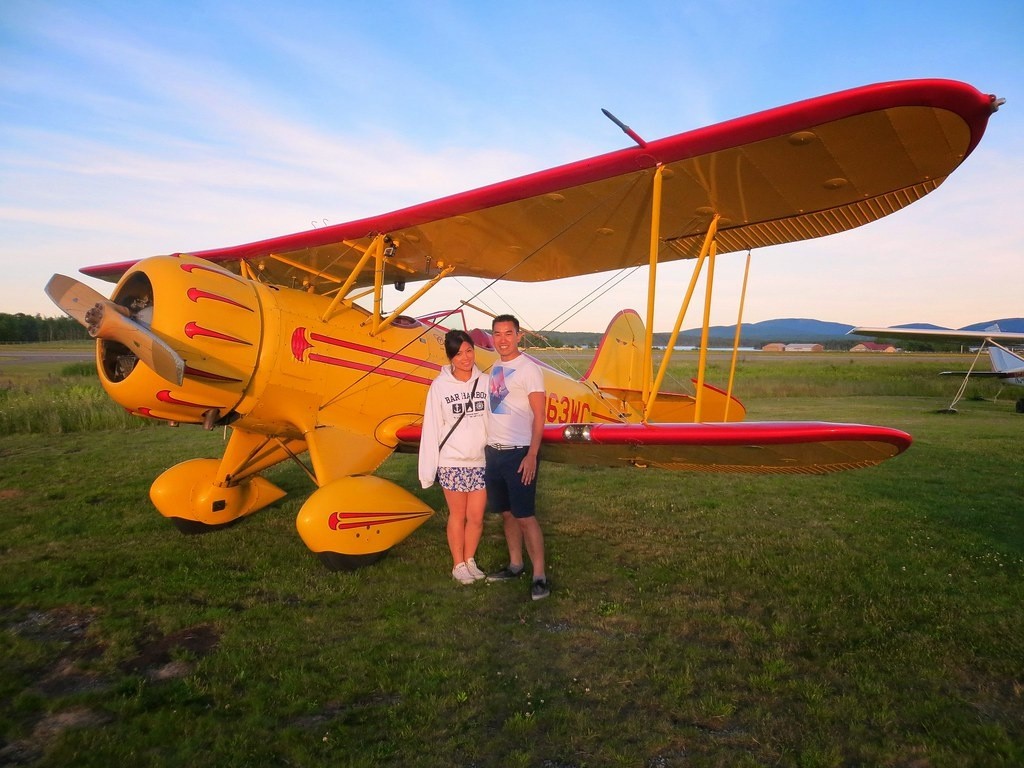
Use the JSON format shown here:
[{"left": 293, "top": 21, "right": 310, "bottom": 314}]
[
  {"left": 486, "top": 568, "right": 526, "bottom": 580},
  {"left": 466, "top": 558, "right": 486, "bottom": 579},
  {"left": 452, "top": 562, "right": 475, "bottom": 584},
  {"left": 532, "top": 580, "right": 550, "bottom": 600}
]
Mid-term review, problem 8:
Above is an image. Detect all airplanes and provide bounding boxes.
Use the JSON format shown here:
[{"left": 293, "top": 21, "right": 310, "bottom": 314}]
[
  {"left": 844, "top": 327, "right": 1024, "bottom": 416},
  {"left": 43, "top": 78, "right": 1006, "bottom": 572}
]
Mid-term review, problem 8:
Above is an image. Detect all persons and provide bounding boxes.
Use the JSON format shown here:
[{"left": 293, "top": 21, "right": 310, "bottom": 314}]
[
  {"left": 485, "top": 314, "right": 551, "bottom": 600},
  {"left": 418, "top": 330, "right": 489, "bottom": 584}
]
